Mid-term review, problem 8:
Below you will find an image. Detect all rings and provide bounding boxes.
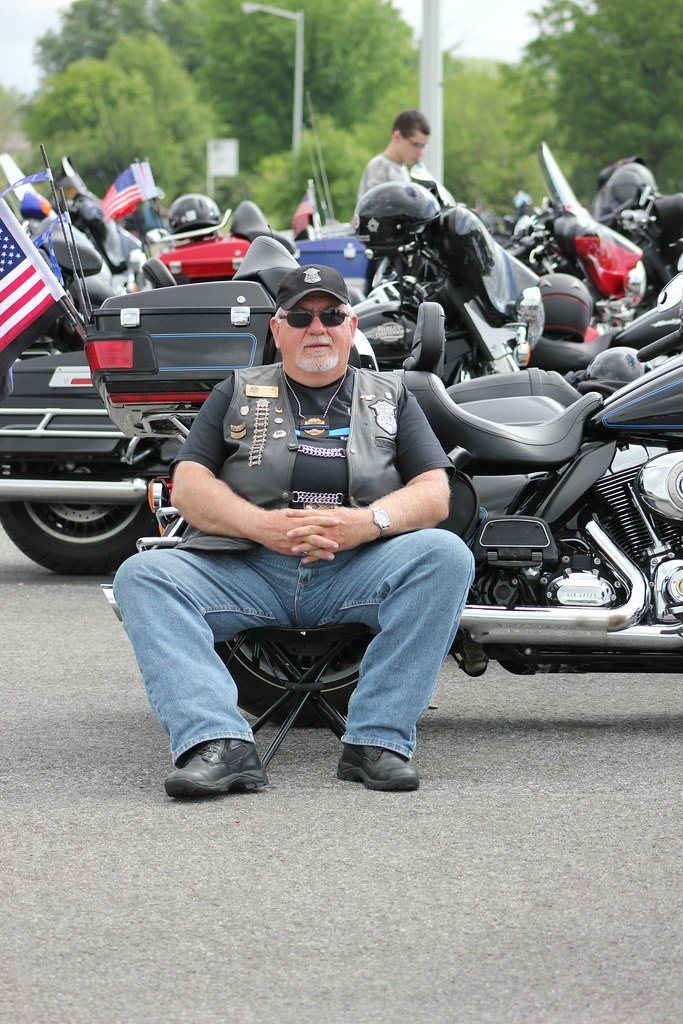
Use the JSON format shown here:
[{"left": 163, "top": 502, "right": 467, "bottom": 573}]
[{"left": 300, "top": 550, "right": 308, "bottom": 558}]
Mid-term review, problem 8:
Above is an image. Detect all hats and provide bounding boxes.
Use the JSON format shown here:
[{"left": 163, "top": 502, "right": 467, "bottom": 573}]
[{"left": 276, "top": 263, "right": 351, "bottom": 310}]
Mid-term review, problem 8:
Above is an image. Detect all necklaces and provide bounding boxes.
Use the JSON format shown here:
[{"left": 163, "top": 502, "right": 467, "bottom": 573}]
[{"left": 282, "top": 371, "right": 347, "bottom": 439}]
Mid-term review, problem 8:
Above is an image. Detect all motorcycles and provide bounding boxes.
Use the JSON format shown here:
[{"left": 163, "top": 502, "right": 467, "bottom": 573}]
[
  {"left": 1, "top": 144, "right": 371, "bottom": 576},
  {"left": 100, "top": 141, "right": 683, "bottom": 726}
]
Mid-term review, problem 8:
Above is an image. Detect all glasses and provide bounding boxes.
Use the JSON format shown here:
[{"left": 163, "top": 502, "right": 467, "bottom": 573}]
[
  {"left": 276, "top": 306, "right": 350, "bottom": 328},
  {"left": 406, "top": 133, "right": 425, "bottom": 148}
]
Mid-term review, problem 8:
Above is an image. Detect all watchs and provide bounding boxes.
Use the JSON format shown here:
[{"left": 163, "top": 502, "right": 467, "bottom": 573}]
[{"left": 366, "top": 505, "right": 390, "bottom": 537}]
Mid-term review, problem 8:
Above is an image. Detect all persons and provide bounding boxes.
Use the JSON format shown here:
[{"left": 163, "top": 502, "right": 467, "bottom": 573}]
[
  {"left": 353, "top": 106, "right": 456, "bottom": 210},
  {"left": 113, "top": 265, "right": 475, "bottom": 796}
]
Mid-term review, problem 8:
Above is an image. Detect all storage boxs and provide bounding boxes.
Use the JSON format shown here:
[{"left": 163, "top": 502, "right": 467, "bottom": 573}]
[
  {"left": 85, "top": 281, "right": 276, "bottom": 436},
  {"left": 295, "top": 235, "right": 368, "bottom": 278},
  {"left": 158, "top": 236, "right": 251, "bottom": 285}
]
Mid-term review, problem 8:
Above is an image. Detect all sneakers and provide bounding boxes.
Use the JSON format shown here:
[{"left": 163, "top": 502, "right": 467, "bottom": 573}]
[
  {"left": 337, "top": 741, "right": 419, "bottom": 791},
  {"left": 164, "top": 739, "right": 269, "bottom": 799}
]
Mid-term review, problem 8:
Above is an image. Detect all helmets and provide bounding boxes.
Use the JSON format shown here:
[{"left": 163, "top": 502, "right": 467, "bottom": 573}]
[
  {"left": 167, "top": 194, "right": 222, "bottom": 235},
  {"left": 353, "top": 180, "right": 441, "bottom": 236},
  {"left": 607, "top": 162, "right": 657, "bottom": 210},
  {"left": 537, "top": 273, "right": 593, "bottom": 342}
]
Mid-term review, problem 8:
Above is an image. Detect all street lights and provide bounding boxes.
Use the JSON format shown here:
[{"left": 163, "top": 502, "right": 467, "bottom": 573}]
[{"left": 240, "top": 3, "right": 304, "bottom": 151}]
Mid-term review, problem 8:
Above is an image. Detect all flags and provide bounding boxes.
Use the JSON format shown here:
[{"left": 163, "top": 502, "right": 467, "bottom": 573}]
[
  {"left": 292, "top": 184, "right": 315, "bottom": 235},
  {"left": 99, "top": 162, "right": 156, "bottom": 220},
  {"left": 0, "top": 199, "right": 66, "bottom": 353}
]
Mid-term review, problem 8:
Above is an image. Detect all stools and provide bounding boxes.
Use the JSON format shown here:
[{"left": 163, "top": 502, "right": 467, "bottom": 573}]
[{"left": 226, "top": 619, "right": 372, "bottom": 774}]
